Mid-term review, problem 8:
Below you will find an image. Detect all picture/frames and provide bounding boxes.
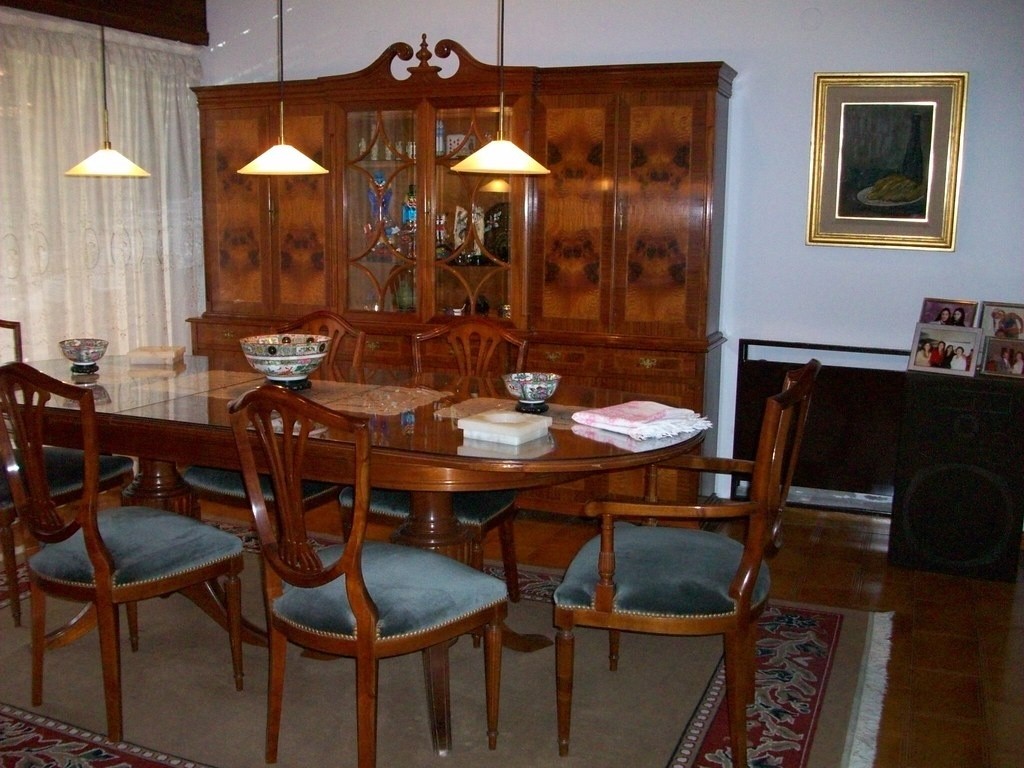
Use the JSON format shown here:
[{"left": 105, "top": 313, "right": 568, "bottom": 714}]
[
  {"left": 907, "top": 323, "right": 982, "bottom": 377},
  {"left": 918, "top": 298, "right": 983, "bottom": 328},
  {"left": 977, "top": 302, "right": 1024, "bottom": 370},
  {"left": 805, "top": 71, "right": 968, "bottom": 252},
  {"left": 979, "top": 336, "right": 1024, "bottom": 378}
]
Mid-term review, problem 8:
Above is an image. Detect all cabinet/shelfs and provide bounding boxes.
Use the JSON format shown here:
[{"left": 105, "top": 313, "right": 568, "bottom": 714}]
[{"left": 187, "top": 60, "right": 737, "bottom": 564}]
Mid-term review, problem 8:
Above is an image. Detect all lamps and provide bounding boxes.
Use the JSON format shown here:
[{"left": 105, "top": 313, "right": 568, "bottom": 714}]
[
  {"left": 479, "top": 179, "right": 510, "bottom": 193},
  {"left": 65, "top": 0, "right": 151, "bottom": 178},
  {"left": 236, "top": 0, "right": 329, "bottom": 176},
  {"left": 450, "top": 0, "right": 550, "bottom": 174}
]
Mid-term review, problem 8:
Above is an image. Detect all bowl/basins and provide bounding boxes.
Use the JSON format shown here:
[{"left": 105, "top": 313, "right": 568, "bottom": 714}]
[
  {"left": 239, "top": 334, "right": 332, "bottom": 382},
  {"left": 502, "top": 373, "right": 562, "bottom": 403},
  {"left": 59, "top": 338, "right": 108, "bottom": 366}
]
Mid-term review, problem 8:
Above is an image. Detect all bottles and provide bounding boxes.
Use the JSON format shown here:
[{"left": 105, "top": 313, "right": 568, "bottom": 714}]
[{"left": 353, "top": 117, "right": 444, "bottom": 310}]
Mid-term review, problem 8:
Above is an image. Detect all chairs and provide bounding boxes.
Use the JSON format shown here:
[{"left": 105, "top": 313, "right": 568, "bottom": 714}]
[{"left": 1, "top": 311, "right": 821, "bottom": 768}]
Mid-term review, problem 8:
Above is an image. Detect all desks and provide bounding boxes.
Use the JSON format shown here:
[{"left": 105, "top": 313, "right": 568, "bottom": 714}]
[{"left": 21, "top": 356, "right": 709, "bottom": 660}]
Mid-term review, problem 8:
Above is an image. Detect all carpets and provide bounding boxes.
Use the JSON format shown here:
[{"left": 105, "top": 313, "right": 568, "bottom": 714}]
[{"left": 1, "top": 511, "right": 896, "bottom": 768}]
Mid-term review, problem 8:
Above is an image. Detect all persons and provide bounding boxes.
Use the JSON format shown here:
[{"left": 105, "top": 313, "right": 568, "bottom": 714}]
[
  {"left": 934, "top": 307, "right": 965, "bottom": 327},
  {"left": 915, "top": 341, "right": 974, "bottom": 371},
  {"left": 991, "top": 308, "right": 1024, "bottom": 338},
  {"left": 998, "top": 351, "right": 1023, "bottom": 375}
]
